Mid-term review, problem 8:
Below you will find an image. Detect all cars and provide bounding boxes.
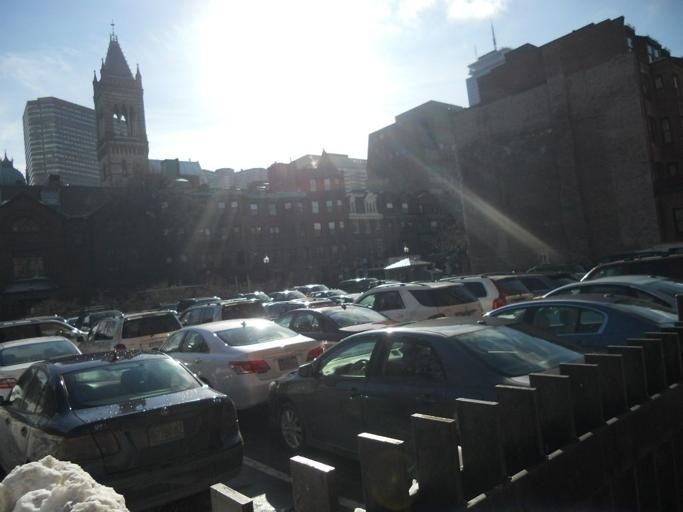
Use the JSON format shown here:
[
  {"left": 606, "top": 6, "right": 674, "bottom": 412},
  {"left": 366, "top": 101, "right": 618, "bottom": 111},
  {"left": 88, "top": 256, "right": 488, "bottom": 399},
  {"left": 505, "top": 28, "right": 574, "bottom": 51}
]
[
  {"left": 150, "top": 317, "right": 325, "bottom": 419},
  {"left": 1, "top": 245, "right": 682, "bottom": 354},
  {"left": 352, "top": 278, "right": 484, "bottom": 327},
  {"left": 516, "top": 273, "right": 681, "bottom": 317},
  {"left": 474, "top": 288, "right": 682, "bottom": 357},
  {"left": 1, "top": 343, "right": 245, "bottom": 510},
  {"left": 273, "top": 303, "right": 417, "bottom": 353},
  {"left": 265, "top": 316, "right": 597, "bottom": 478}
]
